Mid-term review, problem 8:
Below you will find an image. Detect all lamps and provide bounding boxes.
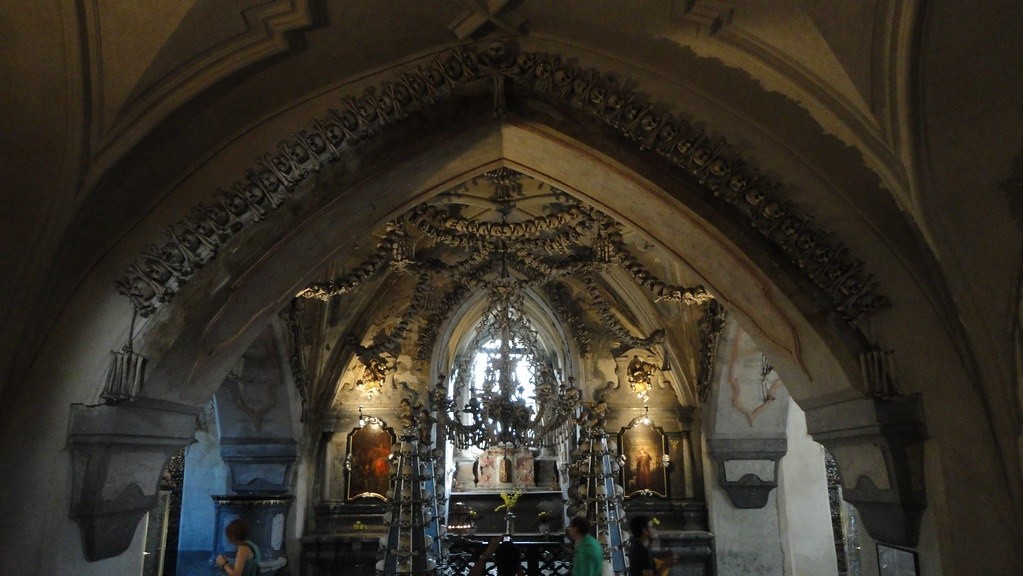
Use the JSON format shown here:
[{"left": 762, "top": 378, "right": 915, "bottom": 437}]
[
  {"left": 413, "top": 238, "right": 608, "bottom": 455},
  {"left": 357, "top": 407, "right": 373, "bottom": 426},
  {"left": 642, "top": 405, "right": 652, "bottom": 426}
]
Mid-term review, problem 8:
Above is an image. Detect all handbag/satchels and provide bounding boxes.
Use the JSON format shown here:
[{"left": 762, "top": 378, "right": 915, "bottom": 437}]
[{"left": 234, "top": 544, "right": 261, "bottom": 576}]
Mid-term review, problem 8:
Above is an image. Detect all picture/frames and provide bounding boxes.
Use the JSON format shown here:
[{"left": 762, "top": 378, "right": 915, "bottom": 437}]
[
  {"left": 617, "top": 415, "right": 669, "bottom": 500},
  {"left": 344, "top": 416, "right": 396, "bottom": 501}
]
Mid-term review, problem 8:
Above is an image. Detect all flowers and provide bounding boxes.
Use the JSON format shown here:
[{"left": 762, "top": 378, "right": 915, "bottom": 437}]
[
  {"left": 465, "top": 507, "right": 477, "bottom": 528},
  {"left": 494, "top": 487, "right": 522, "bottom": 513},
  {"left": 534, "top": 511, "right": 553, "bottom": 526}
]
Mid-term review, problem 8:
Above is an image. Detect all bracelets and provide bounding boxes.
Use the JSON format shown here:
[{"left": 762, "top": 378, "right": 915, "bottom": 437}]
[{"left": 222, "top": 561, "right": 229, "bottom": 569}]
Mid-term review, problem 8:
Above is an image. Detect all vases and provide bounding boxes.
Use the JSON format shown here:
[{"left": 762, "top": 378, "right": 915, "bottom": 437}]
[
  {"left": 538, "top": 522, "right": 549, "bottom": 533},
  {"left": 506, "top": 513, "right": 513, "bottom": 534},
  {"left": 466, "top": 529, "right": 476, "bottom": 534}
]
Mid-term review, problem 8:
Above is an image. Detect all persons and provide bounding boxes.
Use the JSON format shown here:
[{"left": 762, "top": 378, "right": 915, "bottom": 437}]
[
  {"left": 216, "top": 519, "right": 260, "bottom": 576},
  {"left": 568, "top": 515, "right": 604, "bottom": 576},
  {"left": 629, "top": 516, "right": 679, "bottom": 576}
]
